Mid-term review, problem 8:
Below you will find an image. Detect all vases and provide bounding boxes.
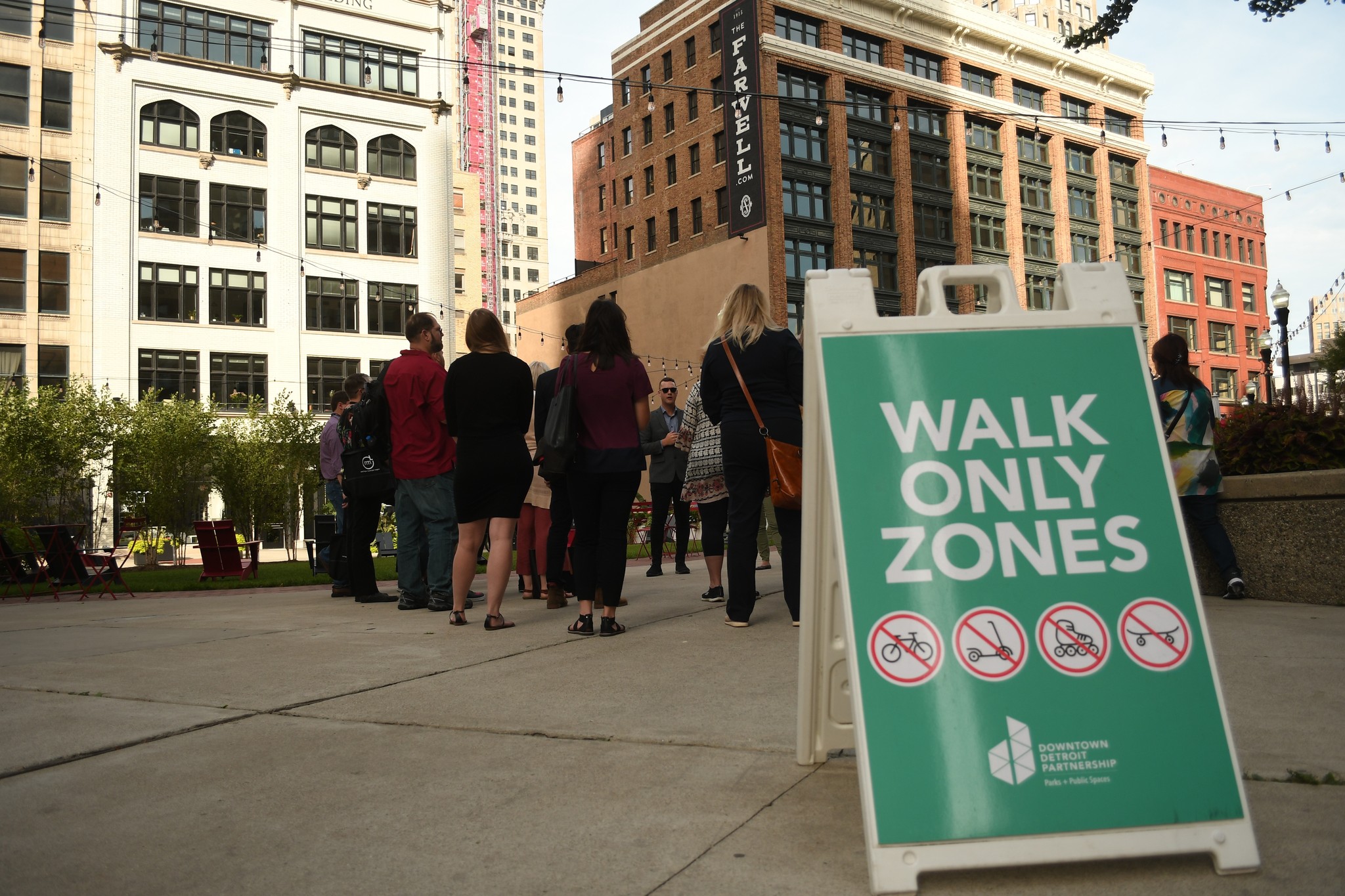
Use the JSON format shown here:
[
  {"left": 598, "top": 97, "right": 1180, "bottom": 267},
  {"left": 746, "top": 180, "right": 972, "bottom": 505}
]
[{"left": 235, "top": 401, "right": 241, "bottom": 408}]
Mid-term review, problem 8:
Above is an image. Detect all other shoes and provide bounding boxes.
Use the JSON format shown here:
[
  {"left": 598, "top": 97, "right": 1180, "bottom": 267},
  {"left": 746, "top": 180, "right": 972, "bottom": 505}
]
[
  {"left": 546, "top": 586, "right": 568, "bottom": 609},
  {"left": 756, "top": 564, "right": 771, "bottom": 570},
  {"left": 331, "top": 585, "right": 354, "bottom": 597},
  {"left": 701, "top": 586, "right": 725, "bottom": 602},
  {"left": 724, "top": 614, "right": 748, "bottom": 628},
  {"left": 355, "top": 592, "right": 399, "bottom": 603},
  {"left": 317, "top": 553, "right": 344, "bottom": 581},
  {"left": 595, "top": 591, "right": 628, "bottom": 607},
  {"left": 755, "top": 591, "right": 761, "bottom": 599},
  {"left": 1222, "top": 573, "right": 1244, "bottom": 600},
  {"left": 792, "top": 620, "right": 799, "bottom": 625}
]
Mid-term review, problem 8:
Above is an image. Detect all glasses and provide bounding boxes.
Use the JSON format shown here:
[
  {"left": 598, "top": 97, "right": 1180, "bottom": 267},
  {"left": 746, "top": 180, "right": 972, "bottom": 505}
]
[
  {"left": 431, "top": 328, "right": 443, "bottom": 334},
  {"left": 659, "top": 387, "right": 677, "bottom": 394}
]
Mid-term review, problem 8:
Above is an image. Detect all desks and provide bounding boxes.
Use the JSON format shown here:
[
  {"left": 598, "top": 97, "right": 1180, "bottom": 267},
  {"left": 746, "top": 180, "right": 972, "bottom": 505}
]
[
  {"left": 661, "top": 504, "right": 688, "bottom": 558},
  {"left": 627, "top": 518, "right": 640, "bottom": 545},
  {"left": 19, "top": 522, "right": 90, "bottom": 602}
]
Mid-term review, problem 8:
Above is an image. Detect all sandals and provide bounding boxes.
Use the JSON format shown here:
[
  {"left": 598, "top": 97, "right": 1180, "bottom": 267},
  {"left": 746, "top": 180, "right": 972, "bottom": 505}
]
[
  {"left": 484, "top": 612, "right": 515, "bottom": 630},
  {"left": 523, "top": 589, "right": 533, "bottom": 599},
  {"left": 599, "top": 616, "right": 626, "bottom": 637},
  {"left": 567, "top": 613, "right": 594, "bottom": 635},
  {"left": 449, "top": 610, "right": 467, "bottom": 625},
  {"left": 540, "top": 588, "right": 573, "bottom": 599}
]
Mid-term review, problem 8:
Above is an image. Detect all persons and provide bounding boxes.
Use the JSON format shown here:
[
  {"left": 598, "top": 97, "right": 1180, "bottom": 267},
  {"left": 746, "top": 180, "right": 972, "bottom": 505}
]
[
  {"left": 555, "top": 297, "right": 654, "bottom": 636},
  {"left": 640, "top": 348, "right": 782, "bottom": 601},
  {"left": 320, "top": 374, "right": 399, "bottom": 604},
  {"left": 700, "top": 284, "right": 804, "bottom": 627},
  {"left": 443, "top": 308, "right": 534, "bottom": 630},
  {"left": 515, "top": 323, "right": 628, "bottom": 608},
  {"left": 382, "top": 312, "right": 485, "bottom": 611},
  {"left": 1144, "top": 333, "right": 1245, "bottom": 599}
]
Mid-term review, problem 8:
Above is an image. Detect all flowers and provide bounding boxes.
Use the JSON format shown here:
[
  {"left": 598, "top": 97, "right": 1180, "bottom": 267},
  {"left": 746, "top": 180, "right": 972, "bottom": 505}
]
[{"left": 229, "top": 391, "right": 247, "bottom": 407}]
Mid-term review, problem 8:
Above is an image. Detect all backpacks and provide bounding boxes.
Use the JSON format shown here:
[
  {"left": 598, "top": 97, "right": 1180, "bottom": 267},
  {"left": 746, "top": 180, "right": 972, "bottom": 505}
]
[{"left": 346, "top": 379, "right": 393, "bottom": 460}]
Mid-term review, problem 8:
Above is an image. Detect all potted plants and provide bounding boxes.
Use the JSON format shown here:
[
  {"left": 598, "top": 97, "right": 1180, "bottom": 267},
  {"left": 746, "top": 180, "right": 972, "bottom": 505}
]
[
  {"left": 232, "top": 314, "right": 243, "bottom": 322},
  {"left": 127, "top": 537, "right": 180, "bottom": 565},
  {"left": 188, "top": 310, "right": 197, "bottom": 320}
]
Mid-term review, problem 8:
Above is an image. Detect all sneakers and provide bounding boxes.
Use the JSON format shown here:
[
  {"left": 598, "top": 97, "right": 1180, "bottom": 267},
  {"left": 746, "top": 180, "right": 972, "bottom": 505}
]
[
  {"left": 466, "top": 590, "right": 485, "bottom": 602},
  {"left": 646, "top": 563, "right": 663, "bottom": 577},
  {"left": 427, "top": 590, "right": 473, "bottom": 611},
  {"left": 675, "top": 562, "right": 690, "bottom": 574},
  {"left": 398, "top": 590, "right": 428, "bottom": 610}
]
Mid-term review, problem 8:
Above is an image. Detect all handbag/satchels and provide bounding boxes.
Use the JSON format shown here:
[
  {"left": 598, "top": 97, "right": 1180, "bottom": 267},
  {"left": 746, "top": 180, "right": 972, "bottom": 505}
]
[
  {"left": 338, "top": 437, "right": 395, "bottom": 506},
  {"left": 762, "top": 434, "right": 802, "bottom": 509},
  {"left": 544, "top": 354, "right": 578, "bottom": 455}
]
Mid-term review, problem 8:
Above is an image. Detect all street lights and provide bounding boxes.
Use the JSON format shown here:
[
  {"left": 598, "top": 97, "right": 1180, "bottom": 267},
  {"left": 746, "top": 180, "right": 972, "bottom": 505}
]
[
  {"left": 1270, "top": 278, "right": 1293, "bottom": 406},
  {"left": 1257, "top": 325, "right": 1273, "bottom": 406}
]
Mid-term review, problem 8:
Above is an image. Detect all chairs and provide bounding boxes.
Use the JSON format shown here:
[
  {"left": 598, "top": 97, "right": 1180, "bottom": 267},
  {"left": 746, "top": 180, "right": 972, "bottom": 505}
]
[
  {"left": 75, "top": 515, "right": 149, "bottom": 602},
  {"left": 375, "top": 531, "right": 398, "bottom": 558},
  {"left": 191, "top": 518, "right": 265, "bottom": 581},
  {"left": 301, "top": 512, "right": 339, "bottom": 578},
  {"left": 626, "top": 500, "right": 703, "bottom": 561},
  {"left": 0, "top": 527, "right": 59, "bottom": 601}
]
